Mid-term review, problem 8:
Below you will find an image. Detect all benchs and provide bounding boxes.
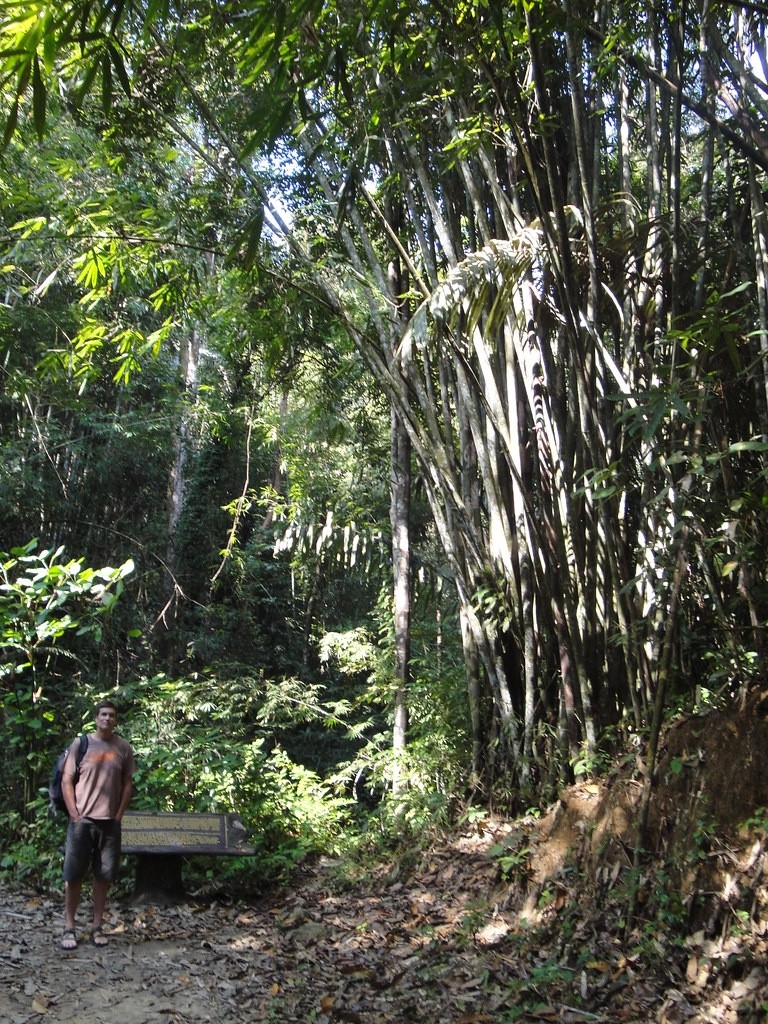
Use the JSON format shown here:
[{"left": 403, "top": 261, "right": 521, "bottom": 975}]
[{"left": 120, "top": 814, "right": 255, "bottom": 905}]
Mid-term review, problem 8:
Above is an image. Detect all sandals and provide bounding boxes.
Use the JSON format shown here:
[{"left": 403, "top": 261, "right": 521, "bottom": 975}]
[
  {"left": 59, "top": 928, "right": 78, "bottom": 950},
  {"left": 90, "top": 927, "right": 109, "bottom": 947}
]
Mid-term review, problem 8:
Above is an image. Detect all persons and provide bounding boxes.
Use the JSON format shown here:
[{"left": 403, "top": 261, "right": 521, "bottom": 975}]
[{"left": 58, "top": 700, "right": 137, "bottom": 950}]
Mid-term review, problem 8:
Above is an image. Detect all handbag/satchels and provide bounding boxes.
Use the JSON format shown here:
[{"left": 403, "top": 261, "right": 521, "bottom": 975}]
[{"left": 49, "top": 735, "right": 89, "bottom": 816}]
